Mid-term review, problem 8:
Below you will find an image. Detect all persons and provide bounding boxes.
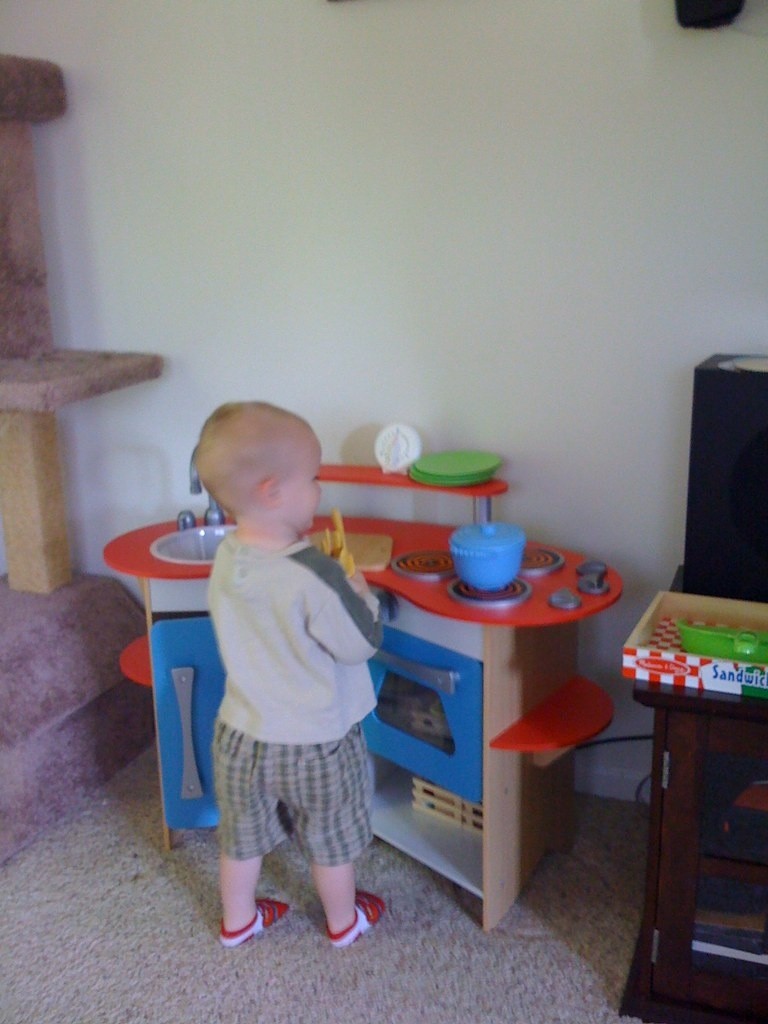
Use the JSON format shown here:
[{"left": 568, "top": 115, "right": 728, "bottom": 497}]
[{"left": 191, "top": 400, "right": 390, "bottom": 949}]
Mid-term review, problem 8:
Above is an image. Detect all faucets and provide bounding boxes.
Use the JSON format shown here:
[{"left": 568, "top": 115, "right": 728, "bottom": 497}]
[{"left": 189, "top": 443, "right": 226, "bottom": 525}]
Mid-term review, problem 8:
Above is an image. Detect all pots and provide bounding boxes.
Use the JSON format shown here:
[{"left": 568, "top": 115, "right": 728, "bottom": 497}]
[
  {"left": 451, "top": 523, "right": 525, "bottom": 593},
  {"left": 673, "top": 619, "right": 768, "bottom": 664}
]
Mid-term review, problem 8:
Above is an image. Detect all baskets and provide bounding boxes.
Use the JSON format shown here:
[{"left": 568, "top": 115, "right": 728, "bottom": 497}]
[{"left": 410, "top": 778, "right": 484, "bottom": 835}]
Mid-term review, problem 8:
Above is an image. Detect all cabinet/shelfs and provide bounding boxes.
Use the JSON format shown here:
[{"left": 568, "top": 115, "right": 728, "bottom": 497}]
[{"left": 617, "top": 679, "right": 768, "bottom": 1024}]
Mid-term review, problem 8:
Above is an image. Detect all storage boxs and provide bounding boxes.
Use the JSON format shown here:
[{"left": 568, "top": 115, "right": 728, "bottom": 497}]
[{"left": 621, "top": 589, "right": 768, "bottom": 700}]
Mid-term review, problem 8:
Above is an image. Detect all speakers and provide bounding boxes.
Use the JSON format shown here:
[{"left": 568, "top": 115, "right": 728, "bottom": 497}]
[{"left": 682, "top": 352, "right": 768, "bottom": 605}]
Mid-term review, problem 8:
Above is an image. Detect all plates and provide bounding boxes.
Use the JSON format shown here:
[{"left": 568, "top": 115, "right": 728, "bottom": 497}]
[{"left": 407, "top": 446, "right": 504, "bottom": 486}]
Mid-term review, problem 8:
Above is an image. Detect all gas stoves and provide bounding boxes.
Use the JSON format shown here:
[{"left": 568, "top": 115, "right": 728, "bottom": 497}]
[{"left": 387, "top": 519, "right": 622, "bottom": 627}]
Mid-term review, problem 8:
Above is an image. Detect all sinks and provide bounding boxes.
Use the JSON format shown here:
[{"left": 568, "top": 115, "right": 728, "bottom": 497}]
[{"left": 150, "top": 525, "right": 236, "bottom": 565}]
